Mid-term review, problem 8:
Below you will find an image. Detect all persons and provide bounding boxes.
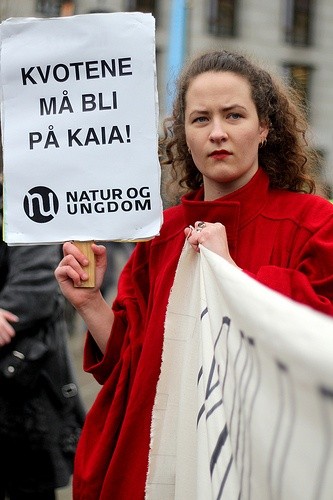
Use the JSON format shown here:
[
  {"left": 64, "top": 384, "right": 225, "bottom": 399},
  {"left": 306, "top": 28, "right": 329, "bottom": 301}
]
[
  {"left": 52, "top": 52, "right": 332, "bottom": 500},
  {"left": 1, "top": 236, "right": 86, "bottom": 493},
  {"left": 0, "top": 309, "right": 57, "bottom": 499}
]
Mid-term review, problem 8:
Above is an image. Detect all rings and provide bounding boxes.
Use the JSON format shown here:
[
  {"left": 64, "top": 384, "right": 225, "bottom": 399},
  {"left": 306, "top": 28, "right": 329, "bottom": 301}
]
[
  {"left": 195, "top": 228, "right": 201, "bottom": 233},
  {"left": 198, "top": 221, "right": 206, "bottom": 228}
]
